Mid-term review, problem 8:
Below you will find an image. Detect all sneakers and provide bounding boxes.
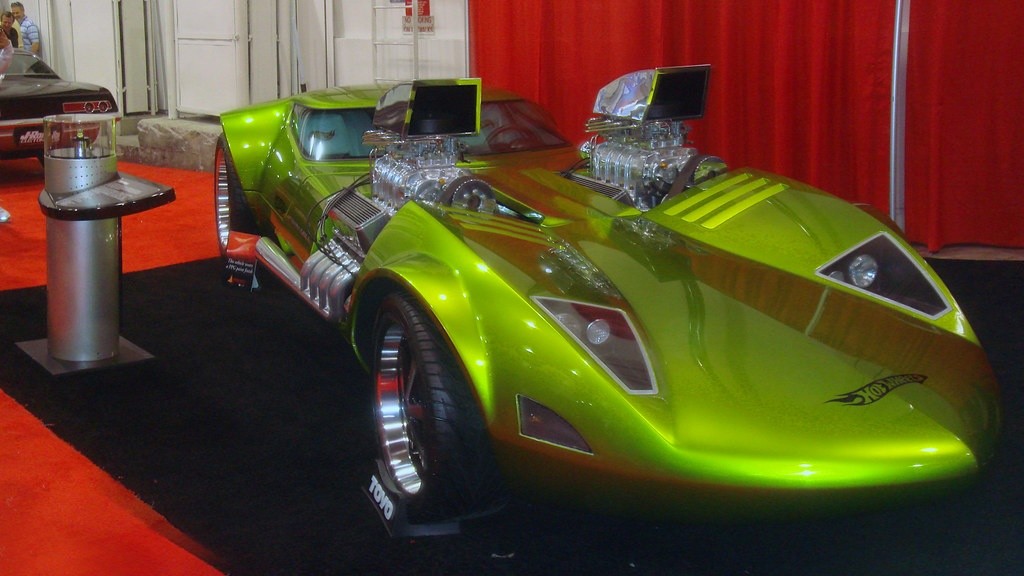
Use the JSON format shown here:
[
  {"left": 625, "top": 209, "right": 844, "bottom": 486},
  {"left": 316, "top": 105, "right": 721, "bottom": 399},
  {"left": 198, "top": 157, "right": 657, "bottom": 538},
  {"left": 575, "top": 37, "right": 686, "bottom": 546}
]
[{"left": 0, "top": 206, "right": 11, "bottom": 223}]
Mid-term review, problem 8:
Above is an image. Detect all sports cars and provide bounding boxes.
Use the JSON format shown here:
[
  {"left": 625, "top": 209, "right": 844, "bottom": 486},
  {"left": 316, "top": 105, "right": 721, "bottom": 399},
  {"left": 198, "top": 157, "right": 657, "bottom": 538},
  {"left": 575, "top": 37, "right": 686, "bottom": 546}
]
[
  {"left": 210, "top": 63, "right": 998, "bottom": 518},
  {"left": 0, "top": 48, "right": 122, "bottom": 168}
]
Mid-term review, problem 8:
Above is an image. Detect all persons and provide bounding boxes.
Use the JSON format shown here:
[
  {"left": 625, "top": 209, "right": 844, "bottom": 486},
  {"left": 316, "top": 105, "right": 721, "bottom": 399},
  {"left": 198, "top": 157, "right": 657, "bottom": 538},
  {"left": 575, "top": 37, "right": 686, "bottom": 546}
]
[
  {"left": 11, "top": 2, "right": 39, "bottom": 53},
  {"left": 0, "top": 28, "right": 15, "bottom": 222},
  {"left": 0, "top": 11, "right": 18, "bottom": 48}
]
